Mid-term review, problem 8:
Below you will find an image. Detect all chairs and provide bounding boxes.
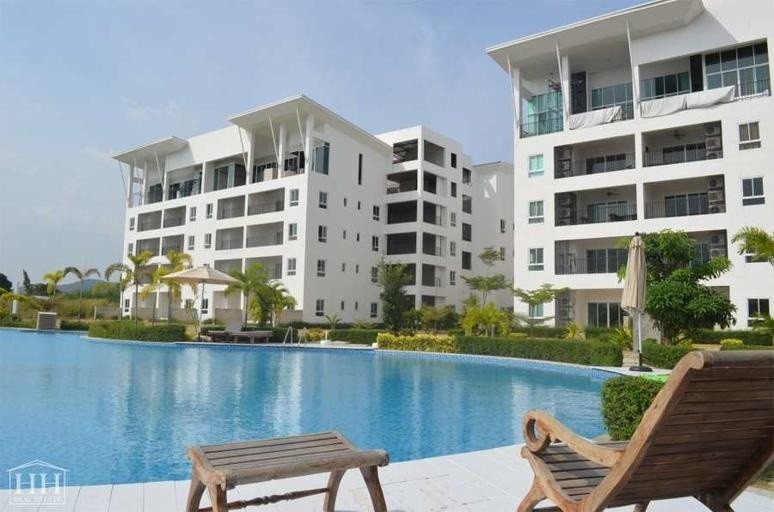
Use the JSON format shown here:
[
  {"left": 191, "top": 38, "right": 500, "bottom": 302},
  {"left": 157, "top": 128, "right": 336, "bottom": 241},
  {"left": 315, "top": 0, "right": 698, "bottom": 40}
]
[{"left": 517, "top": 348, "right": 774, "bottom": 512}]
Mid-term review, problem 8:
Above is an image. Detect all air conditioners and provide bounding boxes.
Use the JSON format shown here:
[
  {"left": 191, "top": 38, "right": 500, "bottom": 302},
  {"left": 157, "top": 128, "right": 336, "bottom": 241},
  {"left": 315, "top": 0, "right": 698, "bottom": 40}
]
[
  {"left": 556, "top": 147, "right": 574, "bottom": 329},
  {"left": 704, "top": 123, "right": 728, "bottom": 262}
]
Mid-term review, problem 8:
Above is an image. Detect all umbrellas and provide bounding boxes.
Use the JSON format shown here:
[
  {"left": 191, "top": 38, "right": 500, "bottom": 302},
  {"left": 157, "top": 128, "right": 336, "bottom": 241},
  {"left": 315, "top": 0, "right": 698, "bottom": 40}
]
[
  {"left": 159, "top": 261, "right": 247, "bottom": 337},
  {"left": 618, "top": 230, "right": 648, "bottom": 367}
]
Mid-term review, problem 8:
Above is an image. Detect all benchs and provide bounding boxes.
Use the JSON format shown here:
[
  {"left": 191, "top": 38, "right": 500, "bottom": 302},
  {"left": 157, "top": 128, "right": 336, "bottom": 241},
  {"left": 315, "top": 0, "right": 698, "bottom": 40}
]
[
  {"left": 186, "top": 430, "right": 390, "bottom": 512},
  {"left": 207, "top": 329, "right": 274, "bottom": 344}
]
